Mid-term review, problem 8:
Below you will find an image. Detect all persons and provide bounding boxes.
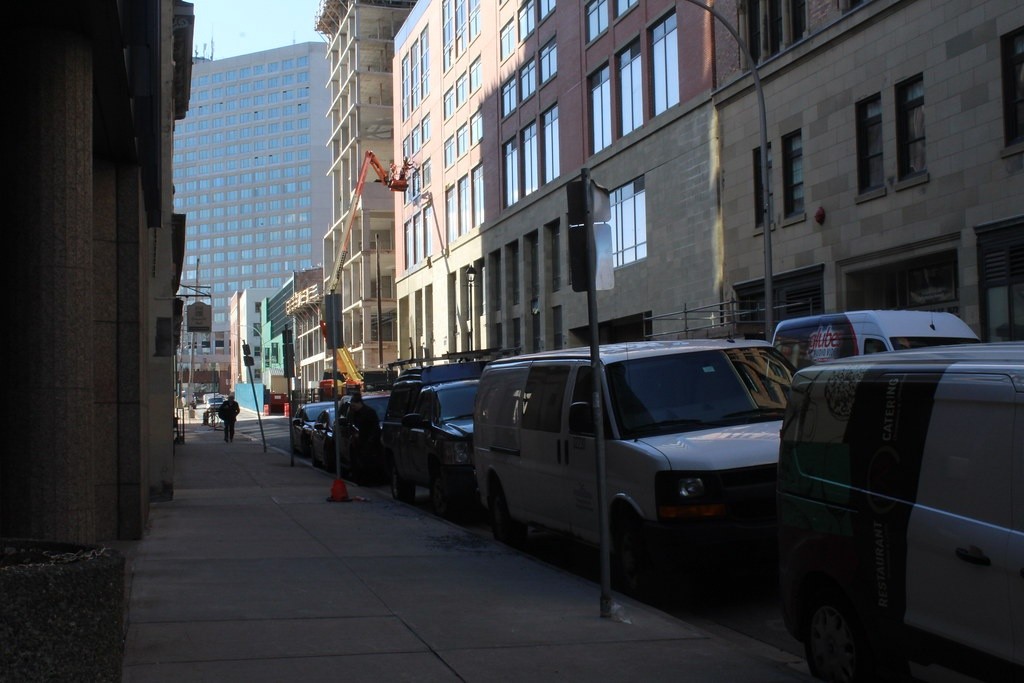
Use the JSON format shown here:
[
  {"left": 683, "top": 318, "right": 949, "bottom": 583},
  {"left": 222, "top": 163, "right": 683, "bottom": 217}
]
[
  {"left": 347, "top": 394, "right": 382, "bottom": 502},
  {"left": 223, "top": 394, "right": 240, "bottom": 442}
]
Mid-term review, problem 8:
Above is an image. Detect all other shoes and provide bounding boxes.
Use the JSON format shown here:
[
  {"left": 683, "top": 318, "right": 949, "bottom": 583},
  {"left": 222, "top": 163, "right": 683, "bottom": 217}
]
[
  {"left": 218, "top": 402, "right": 230, "bottom": 419},
  {"left": 224, "top": 437, "right": 229, "bottom": 443}
]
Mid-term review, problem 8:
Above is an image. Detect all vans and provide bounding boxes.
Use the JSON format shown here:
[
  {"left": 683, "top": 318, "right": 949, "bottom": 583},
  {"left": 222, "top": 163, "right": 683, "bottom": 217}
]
[
  {"left": 779, "top": 341, "right": 1024, "bottom": 682},
  {"left": 769, "top": 307, "right": 982, "bottom": 372},
  {"left": 473, "top": 338, "right": 782, "bottom": 601}
]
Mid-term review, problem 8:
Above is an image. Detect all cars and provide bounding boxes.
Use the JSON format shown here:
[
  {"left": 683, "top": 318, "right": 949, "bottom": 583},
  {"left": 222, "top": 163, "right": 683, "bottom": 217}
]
[
  {"left": 209, "top": 397, "right": 224, "bottom": 411},
  {"left": 292, "top": 402, "right": 334, "bottom": 457},
  {"left": 310, "top": 407, "right": 335, "bottom": 471}
]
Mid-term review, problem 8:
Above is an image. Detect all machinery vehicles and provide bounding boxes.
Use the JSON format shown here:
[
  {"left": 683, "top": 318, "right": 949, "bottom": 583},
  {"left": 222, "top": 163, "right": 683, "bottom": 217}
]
[{"left": 319, "top": 150, "right": 408, "bottom": 394}]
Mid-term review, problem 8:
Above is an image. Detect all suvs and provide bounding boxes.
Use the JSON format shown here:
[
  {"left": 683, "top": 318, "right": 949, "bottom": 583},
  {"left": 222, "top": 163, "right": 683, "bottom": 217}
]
[
  {"left": 380, "top": 347, "right": 520, "bottom": 517},
  {"left": 333, "top": 390, "right": 390, "bottom": 485}
]
